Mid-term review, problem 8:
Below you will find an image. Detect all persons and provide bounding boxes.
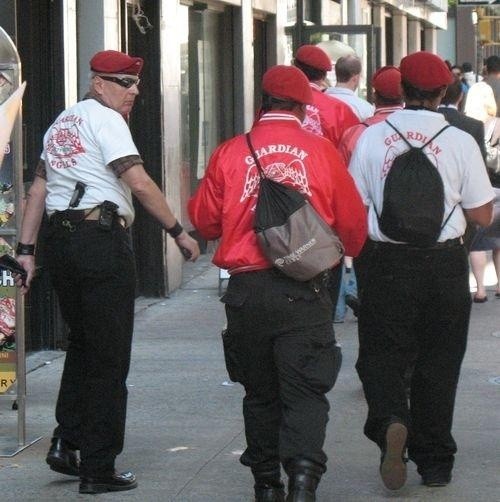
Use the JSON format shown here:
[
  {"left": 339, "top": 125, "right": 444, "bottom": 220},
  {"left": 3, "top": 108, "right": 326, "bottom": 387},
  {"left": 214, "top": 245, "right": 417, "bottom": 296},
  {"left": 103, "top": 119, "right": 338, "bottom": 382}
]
[
  {"left": 10, "top": 49, "right": 199, "bottom": 496},
  {"left": 258, "top": 43, "right": 500, "bottom": 304},
  {"left": 186, "top": 64, "right": 369, "bottom": 502},
  {"left": 345, "top": 51, "right": 497, "bottom": 492}
]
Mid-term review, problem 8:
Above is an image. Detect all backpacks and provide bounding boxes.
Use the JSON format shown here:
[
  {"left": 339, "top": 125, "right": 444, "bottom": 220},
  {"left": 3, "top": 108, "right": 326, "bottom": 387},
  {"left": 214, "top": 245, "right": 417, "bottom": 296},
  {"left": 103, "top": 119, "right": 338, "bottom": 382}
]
[{"left": 383, "top": 121, "right": 449, "bottom": 248}]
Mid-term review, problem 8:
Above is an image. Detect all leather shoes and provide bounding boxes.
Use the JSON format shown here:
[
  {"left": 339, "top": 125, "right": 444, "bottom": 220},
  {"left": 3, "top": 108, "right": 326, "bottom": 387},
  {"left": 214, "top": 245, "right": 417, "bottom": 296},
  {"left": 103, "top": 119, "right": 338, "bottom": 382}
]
[
  {"left": 252, "top": 475, "right": 284, "bottom": 502},
  {"left": 44, "top": 435, "right": 83, "bottom": 476},
  {"left": 418, "top": 459, "right": 454, "bottom": 487},
  {"left": 378, "top": 416, "right": 409, "bottom": 493},
  {"left": 76, "top": 460, "right": 138, "bottom": 496},
  {"left": 286, "top": 458, "right": 323, "bottom": 502}
]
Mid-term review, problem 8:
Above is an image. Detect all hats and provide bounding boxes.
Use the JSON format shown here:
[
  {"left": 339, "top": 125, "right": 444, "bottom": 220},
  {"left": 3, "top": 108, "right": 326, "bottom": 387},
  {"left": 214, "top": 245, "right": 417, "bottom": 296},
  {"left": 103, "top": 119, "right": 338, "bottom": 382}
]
[
  {"left": 261, "top": 63, "right": 316, "bottom": 104},
  {"left": 88, "top": 47, "right": 145, "bottom": 77},
  {"left": 296, "top": 41, "right": 332, "bottom": 74},
  {"left": 370, "top": 65, "right": 404, "bottom": 101},
  {"left": 398, "top": 50, "right": 456, "bottom": 93}
]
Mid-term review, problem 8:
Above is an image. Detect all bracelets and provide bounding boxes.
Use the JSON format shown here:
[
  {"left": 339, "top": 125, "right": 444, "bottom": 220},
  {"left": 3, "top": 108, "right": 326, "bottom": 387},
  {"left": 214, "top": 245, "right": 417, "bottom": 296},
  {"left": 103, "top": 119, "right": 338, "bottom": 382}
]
[
  {"left": 164, "top": 218, "right": 184, "bottom": 239},
  {"left": 16, "top": 241, "right": 35, "bottom": 256}
]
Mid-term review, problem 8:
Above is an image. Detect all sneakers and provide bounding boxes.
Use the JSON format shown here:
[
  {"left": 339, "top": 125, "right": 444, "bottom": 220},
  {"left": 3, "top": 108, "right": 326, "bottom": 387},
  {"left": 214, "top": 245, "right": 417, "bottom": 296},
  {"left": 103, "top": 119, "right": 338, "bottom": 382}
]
[{"left": 343, "top": 293, "right": 360, "bottom": 315}]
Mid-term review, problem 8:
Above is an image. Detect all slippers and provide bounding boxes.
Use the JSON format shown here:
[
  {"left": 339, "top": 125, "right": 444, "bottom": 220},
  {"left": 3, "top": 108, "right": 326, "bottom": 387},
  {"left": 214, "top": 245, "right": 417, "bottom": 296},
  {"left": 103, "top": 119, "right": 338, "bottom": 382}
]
[{"left": 475, "top": 294, "right": 488, "bottom": 304}]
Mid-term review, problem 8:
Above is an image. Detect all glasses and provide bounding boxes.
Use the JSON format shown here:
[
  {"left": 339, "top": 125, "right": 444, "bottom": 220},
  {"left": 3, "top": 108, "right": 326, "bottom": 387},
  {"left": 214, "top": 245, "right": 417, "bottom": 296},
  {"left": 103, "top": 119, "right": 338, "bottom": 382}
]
[{"left": 90, "top": 74, "right": 141, "bottom": 89}]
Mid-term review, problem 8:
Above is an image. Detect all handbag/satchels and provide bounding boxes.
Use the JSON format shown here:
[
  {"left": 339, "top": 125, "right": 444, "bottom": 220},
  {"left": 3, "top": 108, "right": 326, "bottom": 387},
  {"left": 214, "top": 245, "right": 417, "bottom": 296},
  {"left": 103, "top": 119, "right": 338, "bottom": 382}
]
[{"left": 245, "top": 132, "right": 347, "bottom": 283}]
